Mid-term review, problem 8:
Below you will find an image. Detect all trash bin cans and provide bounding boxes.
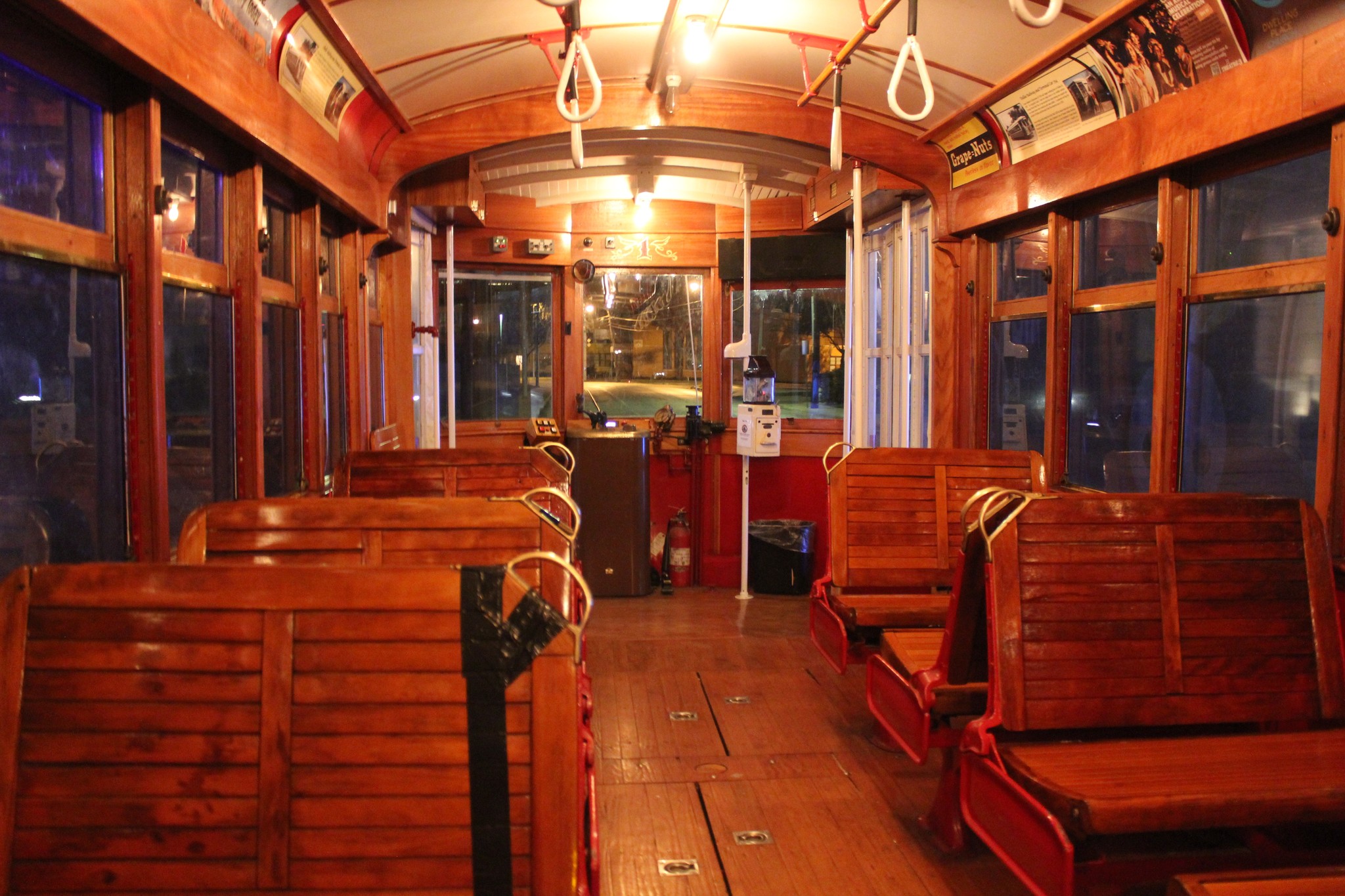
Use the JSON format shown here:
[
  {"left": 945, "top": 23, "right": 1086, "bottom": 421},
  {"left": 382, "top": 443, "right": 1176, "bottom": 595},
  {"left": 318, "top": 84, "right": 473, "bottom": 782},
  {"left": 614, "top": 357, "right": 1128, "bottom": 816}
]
[{"left": 748, "top": 520, "right": 816, "bottom": 596}]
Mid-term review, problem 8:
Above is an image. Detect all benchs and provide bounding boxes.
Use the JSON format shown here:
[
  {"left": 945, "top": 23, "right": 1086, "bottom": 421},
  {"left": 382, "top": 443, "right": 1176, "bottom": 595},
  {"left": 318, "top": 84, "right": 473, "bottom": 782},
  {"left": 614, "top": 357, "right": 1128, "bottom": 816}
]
[
  {"left": 0, "top": 440, "right": 601, "bottom": 896},
  {"left": 863, "top": 485, "right": 1041, "bottom": 858},
  {"left": 807, "top": 441, "right": 1054, "bottom": 675},
  {"left": 959, "top": 486, "right": 1345, "bottom": 896}
]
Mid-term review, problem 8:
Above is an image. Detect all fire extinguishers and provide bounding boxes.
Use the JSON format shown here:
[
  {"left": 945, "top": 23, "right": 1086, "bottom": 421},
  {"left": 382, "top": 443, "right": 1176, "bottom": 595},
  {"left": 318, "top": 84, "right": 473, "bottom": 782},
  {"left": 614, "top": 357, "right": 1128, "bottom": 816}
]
[{"left": 662, "top": 506, "right": 694, "bottom": 595}]
